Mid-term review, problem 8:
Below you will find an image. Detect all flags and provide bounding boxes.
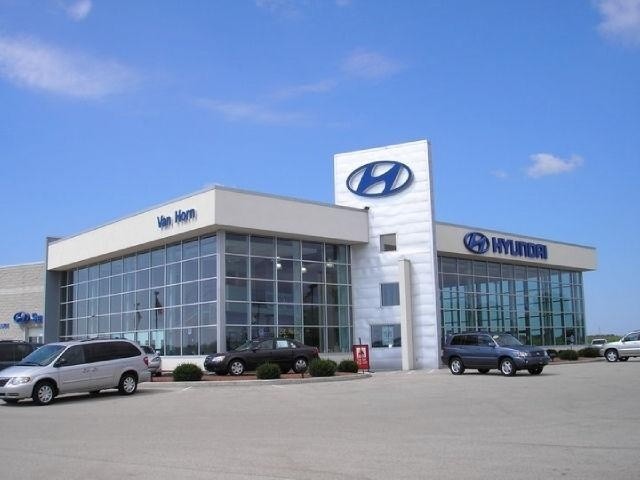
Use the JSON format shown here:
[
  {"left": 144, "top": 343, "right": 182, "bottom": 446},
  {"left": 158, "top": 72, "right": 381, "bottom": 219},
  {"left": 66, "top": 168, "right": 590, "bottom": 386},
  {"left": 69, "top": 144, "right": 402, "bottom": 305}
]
[{"left": 156, "top": 300, "right": 163, "bottom": 314}]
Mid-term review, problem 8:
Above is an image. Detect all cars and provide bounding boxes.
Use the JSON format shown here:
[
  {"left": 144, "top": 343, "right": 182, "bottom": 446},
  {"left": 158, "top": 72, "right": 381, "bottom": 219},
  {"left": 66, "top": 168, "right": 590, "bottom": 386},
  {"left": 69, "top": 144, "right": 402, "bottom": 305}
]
[
  {"left": 141, "top": 345, "right": 161, "bottom": 376},
  {"left": 600, "top": 332, "right": 640, "bottom": 362},
  {"left": 591, "top": 338, "right": 606, "bottom": 350},
  {"left": 204, "top": 337, "right": 318, "bottom": 376}
]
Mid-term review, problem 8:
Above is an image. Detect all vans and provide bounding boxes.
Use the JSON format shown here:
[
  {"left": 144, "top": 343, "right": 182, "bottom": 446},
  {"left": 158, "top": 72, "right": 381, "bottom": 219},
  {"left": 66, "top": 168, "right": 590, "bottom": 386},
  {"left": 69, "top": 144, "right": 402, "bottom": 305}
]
[{"left": 0, "top": 339, "right": 150, "bottom": 405}]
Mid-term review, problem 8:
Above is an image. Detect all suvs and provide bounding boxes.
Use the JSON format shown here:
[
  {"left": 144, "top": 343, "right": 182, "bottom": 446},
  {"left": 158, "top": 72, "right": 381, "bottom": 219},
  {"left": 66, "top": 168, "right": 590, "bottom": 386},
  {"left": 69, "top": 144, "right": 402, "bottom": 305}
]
[{"left": 441, "top": 331, "right": 549, "bottom": 376}]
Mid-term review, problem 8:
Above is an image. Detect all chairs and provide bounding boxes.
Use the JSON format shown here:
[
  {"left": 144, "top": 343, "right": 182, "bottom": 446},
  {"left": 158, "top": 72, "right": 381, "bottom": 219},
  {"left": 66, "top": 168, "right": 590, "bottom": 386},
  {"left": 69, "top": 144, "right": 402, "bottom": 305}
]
[{"left": 55, "top": 349, "right": 89, "bottom": 362}]
